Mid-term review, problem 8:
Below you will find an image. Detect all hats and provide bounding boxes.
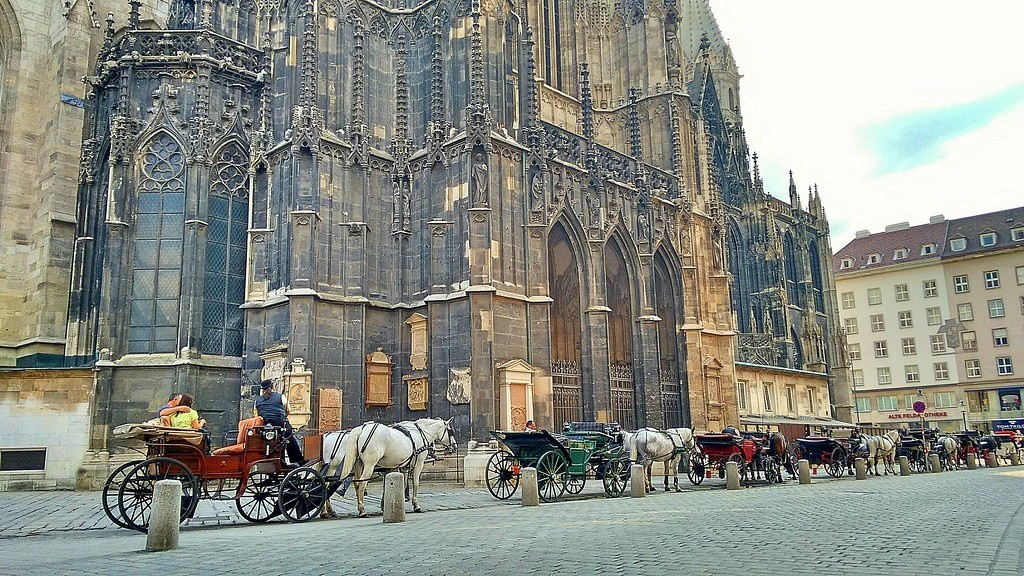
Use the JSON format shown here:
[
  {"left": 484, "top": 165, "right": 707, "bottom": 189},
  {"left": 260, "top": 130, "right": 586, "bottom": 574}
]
[{"left": 259, "top": 379, "right": 274, "bottom": 389}]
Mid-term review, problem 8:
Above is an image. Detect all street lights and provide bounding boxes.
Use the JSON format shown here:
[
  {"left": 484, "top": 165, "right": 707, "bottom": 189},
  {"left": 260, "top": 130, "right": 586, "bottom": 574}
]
[
  {"left": 958, "top": 399, "right": 969, "bottom": 433},
  {"left": 845, "top": 358, "right": 860, "bottom": 427}
]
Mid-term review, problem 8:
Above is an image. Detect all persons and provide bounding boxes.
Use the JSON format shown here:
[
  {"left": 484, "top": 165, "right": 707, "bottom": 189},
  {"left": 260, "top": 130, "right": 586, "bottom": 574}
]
[
  {"left": 721, "top": 424, "right": 740, "bottom": 437},
  {"left": 403, "top": 182, "right": 410, "bottom": 215},
  {"left": 471, "top": 153, "right": 487, "bottom": 204},
  {"left": 391, "top": 181, "right": 400, "bottom": 214},
  {"left": 157, "top": 392, "right": 190, "bottom": 418},
  {"left": 524, "top": 419, "right": 536, "bottom": 432},
  {"left": 169, "top": 394, "right": 212, "bottom": 456},
  {"left": 254, "top": 379, "right": 309, "bottom": 468}
]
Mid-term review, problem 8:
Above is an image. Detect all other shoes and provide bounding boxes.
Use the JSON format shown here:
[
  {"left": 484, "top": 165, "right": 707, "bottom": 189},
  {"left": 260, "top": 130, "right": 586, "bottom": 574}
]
[{"left": 299, "top": 459, "right": 309, "bottom": 464}]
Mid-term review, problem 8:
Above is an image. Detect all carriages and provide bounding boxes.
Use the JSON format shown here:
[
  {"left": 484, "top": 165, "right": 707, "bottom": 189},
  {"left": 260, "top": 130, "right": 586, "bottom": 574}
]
[
  {"left": 793, "top": 428, "right": 901, "bottom": 478},
  {"left": 102, "top": 415, "right": 458, "bottom": 534},
  {"left": 686, "top": 429, "right": 796, "bottom": 486},
  {"left": 899, "top": 430, "right": 1024, "bottom": 474},
  {"left": 485, "top": 421, "right": 701, "bottom": 503}
]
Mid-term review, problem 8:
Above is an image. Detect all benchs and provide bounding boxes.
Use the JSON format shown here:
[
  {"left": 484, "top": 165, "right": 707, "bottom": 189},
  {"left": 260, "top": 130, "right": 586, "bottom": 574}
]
[
  {"left": 565, "top": 436, "right": 598, "bottom": 463},
  {"left": 488, "top": 430, "right": 571, "bottom": 464},
  {"left": 212, "top": 416, "right": 264, "bottom": 455},
  {"left": 741, "top": 432, "right": 763, "bottom": 462},
  {"left": 696, "top": 435, "right": 744, "bottom": 455},
  {"left": 149, "top": 416, "right": 205, "bottom": 455}
]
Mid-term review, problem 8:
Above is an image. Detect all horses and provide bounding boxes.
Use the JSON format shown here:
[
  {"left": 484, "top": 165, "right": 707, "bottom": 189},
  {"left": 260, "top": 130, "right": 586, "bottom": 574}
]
[
  {"left": 620, "top": 425, "right": 699, "bottom": 493},
  {"left": 974, "top": 435, "right": 1007, "bottom": 466},
  {"left": 744, "top": 432, "right": 798, "bottom": 483},
  {"left": 848, "top": 428, "right": 901, "bottom": 477},
  {"left": 934, "top": 433, "right": 962, "bottom": 471},
  {"left": 320, "top": 416, "right": 458, "bottom": 518}
]
